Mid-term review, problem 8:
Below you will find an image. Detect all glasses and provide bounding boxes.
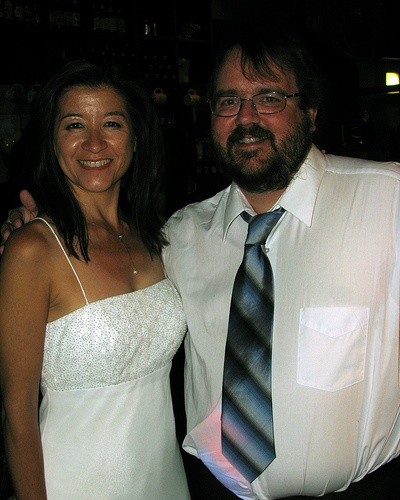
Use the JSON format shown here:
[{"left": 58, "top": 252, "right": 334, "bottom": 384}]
[{"left": 206, "top": 90, "right": 300, "bottom": 117}]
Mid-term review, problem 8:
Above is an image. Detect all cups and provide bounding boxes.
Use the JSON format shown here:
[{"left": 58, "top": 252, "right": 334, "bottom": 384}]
[
  {"left": 177, "top": 63, "right": 190, "bottom": 84},
  {"left": 151, "top": 87, "right": 166, "bottom": 103},
  {"left": 183, "top": 89, "right": 203, "bottom": 107}
]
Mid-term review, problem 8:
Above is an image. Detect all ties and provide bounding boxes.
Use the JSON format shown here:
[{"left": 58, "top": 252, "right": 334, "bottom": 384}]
[{"left": 219, "top": 207, "right": 286, "bottom": 485}]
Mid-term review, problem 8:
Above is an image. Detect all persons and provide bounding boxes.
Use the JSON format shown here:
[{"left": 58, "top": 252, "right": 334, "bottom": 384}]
[
  {"left": 0, "top": 33, "right": 400, "bottom": 500},
  {"left": 0, "top": 61, "right": 191, "bottom": 500}
]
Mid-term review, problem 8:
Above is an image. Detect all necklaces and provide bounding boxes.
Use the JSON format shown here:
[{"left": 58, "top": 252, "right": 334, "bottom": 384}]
[{"left": 87, "top": 222, "right": 137, "bottom": 274}]
